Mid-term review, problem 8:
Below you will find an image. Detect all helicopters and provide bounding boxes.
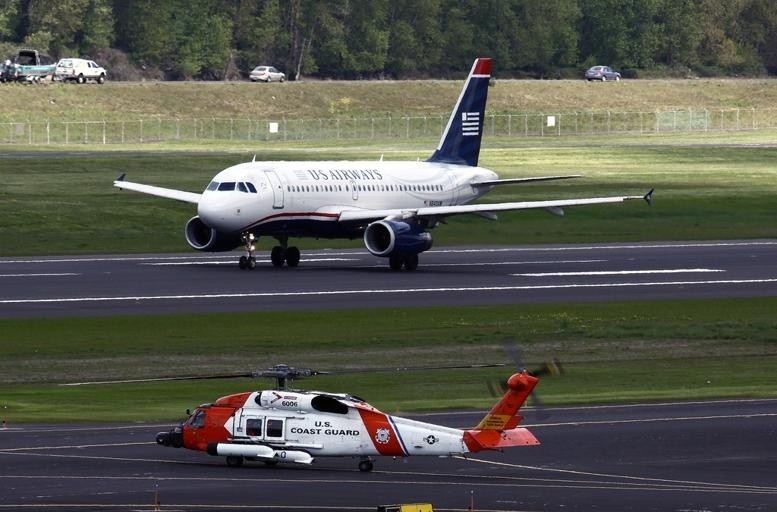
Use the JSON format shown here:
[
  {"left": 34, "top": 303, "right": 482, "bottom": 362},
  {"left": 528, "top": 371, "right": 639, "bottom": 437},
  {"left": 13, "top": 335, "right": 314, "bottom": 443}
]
[{"left": 56, "top": 339, "right": 564, "bottom": 477}]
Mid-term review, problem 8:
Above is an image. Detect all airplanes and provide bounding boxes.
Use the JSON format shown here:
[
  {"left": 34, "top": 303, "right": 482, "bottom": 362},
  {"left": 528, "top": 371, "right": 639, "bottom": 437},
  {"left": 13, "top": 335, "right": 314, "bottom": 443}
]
[{"left": 109, "top": 55, "right": 655, "bottom": 271}]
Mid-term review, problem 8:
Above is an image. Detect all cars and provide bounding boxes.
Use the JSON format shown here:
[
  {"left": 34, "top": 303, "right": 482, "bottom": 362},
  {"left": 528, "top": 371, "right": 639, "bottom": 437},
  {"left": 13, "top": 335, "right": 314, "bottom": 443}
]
[
  {"left": 0, "top": 49, "right": 57, "bottom": 82},
  {"left": 583, "top": 65, "right": 621, "bottom": 83},
  {"left": 247, "top": 65, "right": 286, "bottom": 83}
]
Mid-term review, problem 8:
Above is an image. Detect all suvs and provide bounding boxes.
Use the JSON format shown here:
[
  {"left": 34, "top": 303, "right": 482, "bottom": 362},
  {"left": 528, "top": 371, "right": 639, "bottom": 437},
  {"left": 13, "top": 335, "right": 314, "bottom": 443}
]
[{"left": 54, "top": 58, "right": 107, "bottom": 85}]
